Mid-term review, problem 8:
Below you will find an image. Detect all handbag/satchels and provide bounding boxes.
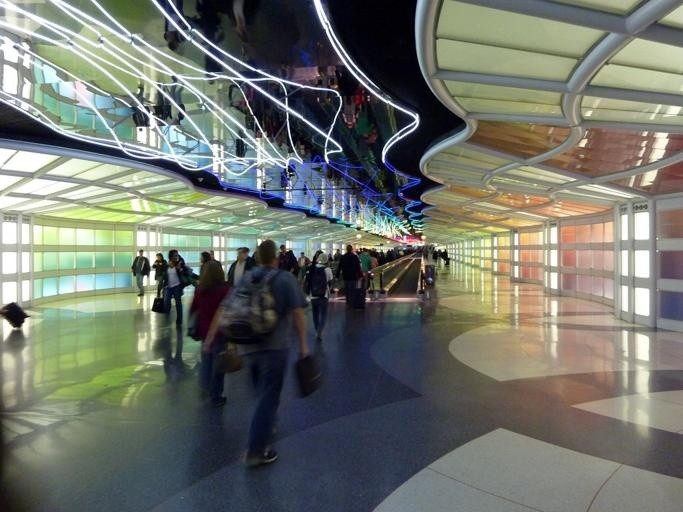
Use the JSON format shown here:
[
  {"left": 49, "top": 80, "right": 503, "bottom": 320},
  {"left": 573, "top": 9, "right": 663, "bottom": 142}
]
[
  {"left": 292, "top": 356, "right": 326, "bottom": 398},
  {"left": 150, "top": 296, "right": 167, "bottom": 314},
  {"left": 214, "top": 350, "right": 246, "bottom": 374}
]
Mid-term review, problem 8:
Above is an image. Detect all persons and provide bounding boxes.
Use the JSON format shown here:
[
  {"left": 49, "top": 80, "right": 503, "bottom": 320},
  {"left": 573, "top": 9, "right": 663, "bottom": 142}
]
[
  {"left": 132, "top": 246, "right": 256, "bottom": 327},
  {"left": 187, "top": 260, "right": 234, "bottom": 413},
  {"left": 201, "top": 239, "right": 310, "bottom": 468},
  {"left": 277, "top": 244, "right": 452, "bottom": 340},
  {"left": 130, "top": 66, "right": 379, "bottom": 216}
]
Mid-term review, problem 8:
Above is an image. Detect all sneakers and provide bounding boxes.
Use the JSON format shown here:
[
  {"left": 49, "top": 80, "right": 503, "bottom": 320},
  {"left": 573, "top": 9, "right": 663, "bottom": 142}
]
[
  {"left": 199, "top": 392, "right": 227, "bottom": 407},
  {"left": 242, "top": 448, "right": 277, "bottom": 467}
]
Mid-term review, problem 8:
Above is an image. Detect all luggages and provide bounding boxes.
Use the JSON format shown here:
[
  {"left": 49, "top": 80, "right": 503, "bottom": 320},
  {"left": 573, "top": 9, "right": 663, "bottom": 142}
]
[
  {"left": 1, "top": 301, "right": 30, "bottom": 328},
  {"left": 348, "top": 287, "right": 366, "bottom": 312}
]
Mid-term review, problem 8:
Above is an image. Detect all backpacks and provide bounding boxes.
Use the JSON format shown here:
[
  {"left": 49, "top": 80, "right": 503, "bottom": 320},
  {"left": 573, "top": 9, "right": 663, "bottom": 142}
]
[
  {"left": 220, "top": 269, "right": 284, "bottom": 345},
  {"left": 307, "top": 265, "right": 329, "bottom": 297}
]
